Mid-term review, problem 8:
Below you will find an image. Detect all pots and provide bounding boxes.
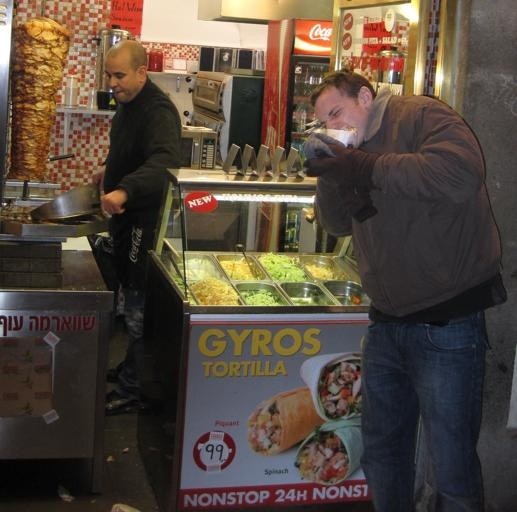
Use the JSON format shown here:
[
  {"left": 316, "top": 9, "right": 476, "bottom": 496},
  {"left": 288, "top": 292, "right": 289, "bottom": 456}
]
[{"left": 371, "top": 45, "right": 408, "bottom": 84}]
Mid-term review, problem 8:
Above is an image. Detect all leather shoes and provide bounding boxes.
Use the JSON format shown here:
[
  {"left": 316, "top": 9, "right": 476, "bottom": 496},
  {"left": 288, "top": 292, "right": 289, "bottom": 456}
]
[
  {"left": 107, "top": 367, "right": 119, "bottom": 383},
  {"left": 105, "top": 388, "right": 141, "bottom": 414}
]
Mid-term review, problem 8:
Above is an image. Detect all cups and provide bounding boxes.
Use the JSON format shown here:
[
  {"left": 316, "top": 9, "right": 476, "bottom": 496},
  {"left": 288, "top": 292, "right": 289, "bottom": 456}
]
[
  {"left": 87, "top": 87, "right": 98, "bottom": 110},
  {"left": 147, "top": 48, "right": 163, "bottom": 72}
]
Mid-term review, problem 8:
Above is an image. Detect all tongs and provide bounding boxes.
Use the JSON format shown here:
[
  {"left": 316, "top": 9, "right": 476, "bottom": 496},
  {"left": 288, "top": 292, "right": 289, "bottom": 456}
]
[{"left": 230, "top": 243, "right": 258, "bottom": 280}]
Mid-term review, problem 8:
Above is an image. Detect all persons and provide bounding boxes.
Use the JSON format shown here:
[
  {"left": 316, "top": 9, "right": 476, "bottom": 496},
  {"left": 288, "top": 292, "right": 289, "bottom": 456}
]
[
  {"left": 301, "top": 69, "right": 506, "bottom": 512},
  {"left": 92, "top": 40, "right": 182, "bottom": 416}
]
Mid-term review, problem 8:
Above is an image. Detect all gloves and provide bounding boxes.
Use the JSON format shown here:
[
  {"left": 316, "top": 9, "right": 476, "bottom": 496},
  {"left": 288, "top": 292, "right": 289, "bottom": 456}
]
[
  {"left": 339, "top": 143, "right": 378, "bottom": 222},
  {"left": 303, "top": 132, "right": 375, "bottom": 187}
]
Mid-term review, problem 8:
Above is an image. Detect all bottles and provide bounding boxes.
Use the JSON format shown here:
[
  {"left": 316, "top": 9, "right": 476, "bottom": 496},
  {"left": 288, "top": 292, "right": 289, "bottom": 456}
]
[
  {"left": 65, "top": 76, "right": 78, "bottom": 108},
  {"left": 293, "top": 62, "right": 329, "bottom": 132}
]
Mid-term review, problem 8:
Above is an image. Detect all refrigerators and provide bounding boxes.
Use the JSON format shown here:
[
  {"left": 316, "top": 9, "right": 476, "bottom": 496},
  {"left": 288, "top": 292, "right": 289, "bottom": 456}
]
[{"left": 255, "top": 17, "right": 337, "bottom": 253}]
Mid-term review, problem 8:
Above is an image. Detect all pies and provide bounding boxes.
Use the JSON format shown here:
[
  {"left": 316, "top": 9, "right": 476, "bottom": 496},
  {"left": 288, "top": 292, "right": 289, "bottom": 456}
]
[
  {"left": 294, "top": 416, "right": 376, "bottom": 484},
  {"left": 298, "top": 353, "right": 366, "bottom": 420},
  {"left": 246, "top": 389, "right": 320, "bottom": 454}
]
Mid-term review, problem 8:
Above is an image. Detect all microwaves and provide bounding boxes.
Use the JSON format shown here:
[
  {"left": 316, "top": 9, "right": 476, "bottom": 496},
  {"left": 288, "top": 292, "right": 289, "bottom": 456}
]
[{"left": 178, "top": 125, "right": 218, "bottom": 171}]
